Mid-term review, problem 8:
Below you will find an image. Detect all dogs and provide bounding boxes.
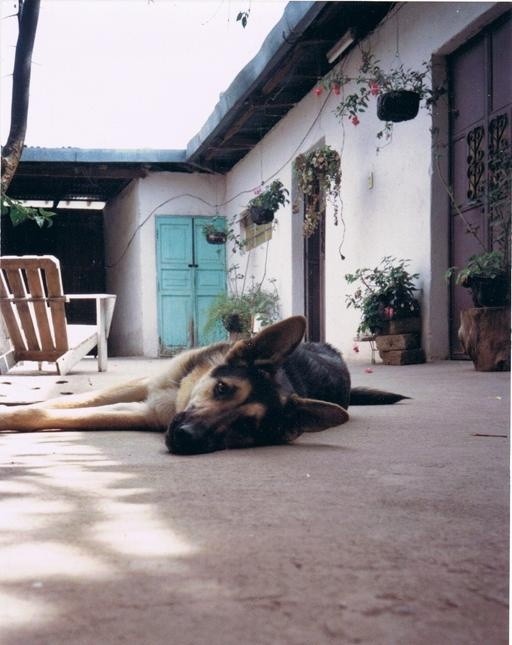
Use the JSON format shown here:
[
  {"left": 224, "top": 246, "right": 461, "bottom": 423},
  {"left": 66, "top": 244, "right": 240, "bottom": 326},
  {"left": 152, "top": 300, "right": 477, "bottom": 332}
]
[{"left": 0, "top": 315, "right": 415, "bottom": 455}]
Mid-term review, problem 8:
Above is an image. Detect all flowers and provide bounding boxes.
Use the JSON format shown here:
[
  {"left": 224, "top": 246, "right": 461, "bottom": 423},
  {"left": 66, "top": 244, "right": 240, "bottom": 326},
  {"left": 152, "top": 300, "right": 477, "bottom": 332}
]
[
  {"left": 343, "top": 255, "right": 423, "bottom": 373},
  {"left": 312, "top": 48, "right": 459, "bottom": 139}
]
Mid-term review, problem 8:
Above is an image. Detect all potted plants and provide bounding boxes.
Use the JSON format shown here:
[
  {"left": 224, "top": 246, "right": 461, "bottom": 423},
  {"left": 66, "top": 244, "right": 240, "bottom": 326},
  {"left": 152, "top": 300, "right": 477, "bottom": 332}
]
[
  {"left": 246, "top": 179, "right": 290, "bottom": 225},
  {"left": 444, "top": 247, "right": 512, "bottom": 307},
  {"left": 195, "top": 212, "right": 247, "bottom": 253},
  {"left": 292, "top": 144, "right": 345, "bottom": 259},
  {"left": 198, "top": 266, "right": 281, "bottom": 347}
]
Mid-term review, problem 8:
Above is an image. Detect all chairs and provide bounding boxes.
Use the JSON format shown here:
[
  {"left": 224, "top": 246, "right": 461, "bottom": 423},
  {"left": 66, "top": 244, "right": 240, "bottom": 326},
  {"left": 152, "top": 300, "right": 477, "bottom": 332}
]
[{"left": 0, "top": 255, "right": 118, "bottom": 377}]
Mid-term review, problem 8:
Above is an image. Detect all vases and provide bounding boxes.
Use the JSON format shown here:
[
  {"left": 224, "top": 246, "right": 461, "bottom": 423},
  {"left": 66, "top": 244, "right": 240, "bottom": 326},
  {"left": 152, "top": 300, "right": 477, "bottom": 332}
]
[
  {"left": 377, "top": 308, "right": 422, "bottom": 334},
  {"left": 376, "top": 89, "right": 420, "bottom": 123}
]
